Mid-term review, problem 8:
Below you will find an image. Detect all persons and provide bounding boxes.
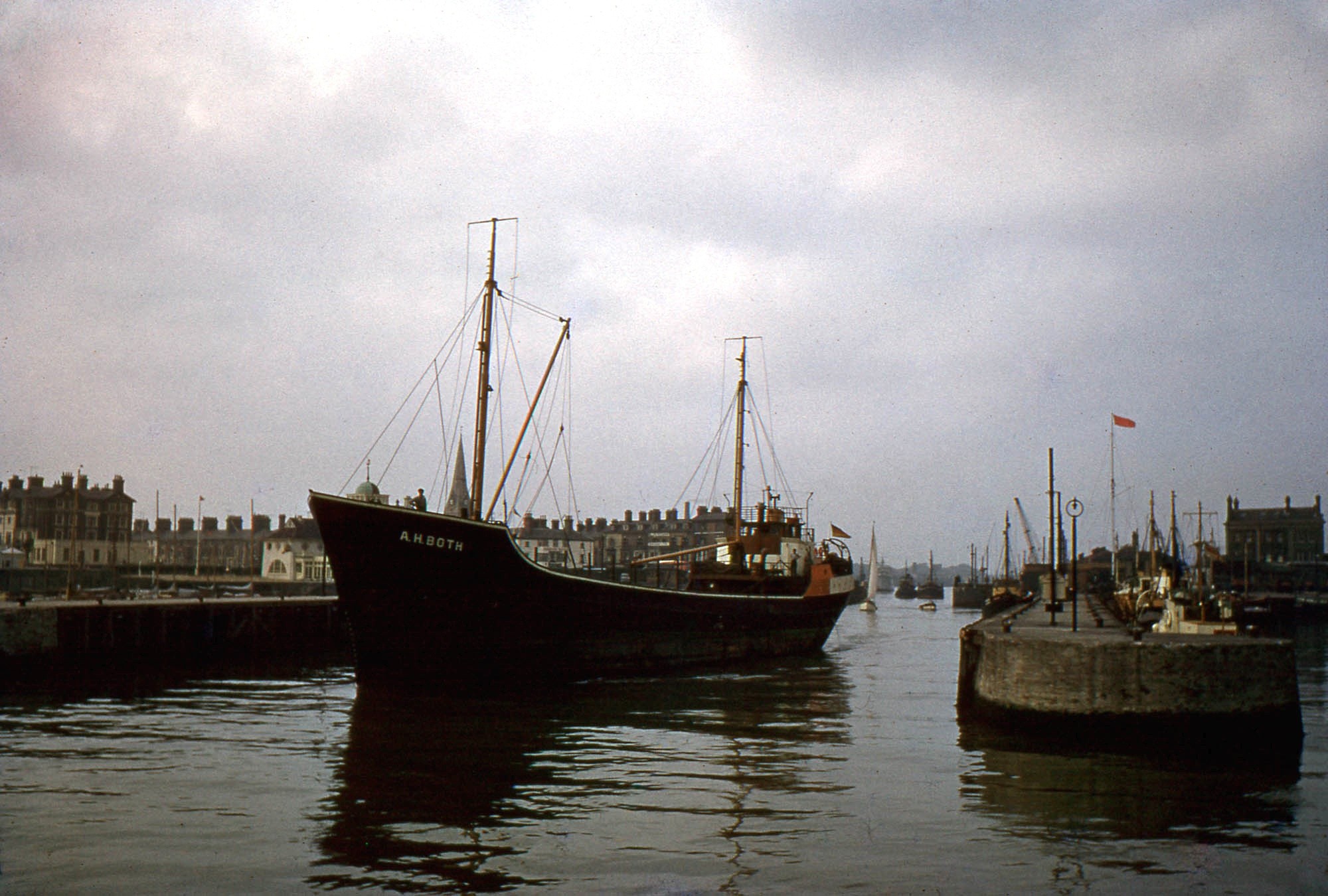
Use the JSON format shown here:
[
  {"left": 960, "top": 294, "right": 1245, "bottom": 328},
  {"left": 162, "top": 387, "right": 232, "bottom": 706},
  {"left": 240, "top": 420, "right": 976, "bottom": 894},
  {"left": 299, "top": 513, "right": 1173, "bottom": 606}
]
[{"left": 789, "top": 548, "right": 800, "bottom": 575}]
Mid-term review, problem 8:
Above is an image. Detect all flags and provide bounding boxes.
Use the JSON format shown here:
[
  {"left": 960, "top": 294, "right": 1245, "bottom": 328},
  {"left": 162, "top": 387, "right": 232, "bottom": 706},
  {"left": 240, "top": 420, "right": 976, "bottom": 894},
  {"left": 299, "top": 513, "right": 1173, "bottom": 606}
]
[
  {"left": 1112, "top": 412, "right": 1136, "bottom": 428},
  {"left": 829, "top": 523, "right": 851, "bottom": 539}
]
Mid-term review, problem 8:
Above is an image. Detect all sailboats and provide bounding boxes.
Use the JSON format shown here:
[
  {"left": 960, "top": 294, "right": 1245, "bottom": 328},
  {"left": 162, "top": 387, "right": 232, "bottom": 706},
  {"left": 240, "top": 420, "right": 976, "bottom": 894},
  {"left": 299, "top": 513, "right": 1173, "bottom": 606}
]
[{"left": 859, "top": 520, "right": 879, "bottom": 612}]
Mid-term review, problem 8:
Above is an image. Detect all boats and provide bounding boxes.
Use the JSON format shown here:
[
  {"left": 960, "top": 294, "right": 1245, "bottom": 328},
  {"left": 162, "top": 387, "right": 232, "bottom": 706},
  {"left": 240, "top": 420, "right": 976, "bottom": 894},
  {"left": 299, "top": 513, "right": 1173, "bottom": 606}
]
[
  {"left": 917, "top": 550, "right": 945, "bottom": 600},
  {"left": 895, "top": 560, "right": 919, "bottom": 599},
  {"left": 305, "top": 216, "right": 851, "bottom": 684},
  {"left": 952, "top": 542, "right": 993, "bottom": 610},
  {"left": 919, "top": 600, "right": 936, "bottom": 612}
]
